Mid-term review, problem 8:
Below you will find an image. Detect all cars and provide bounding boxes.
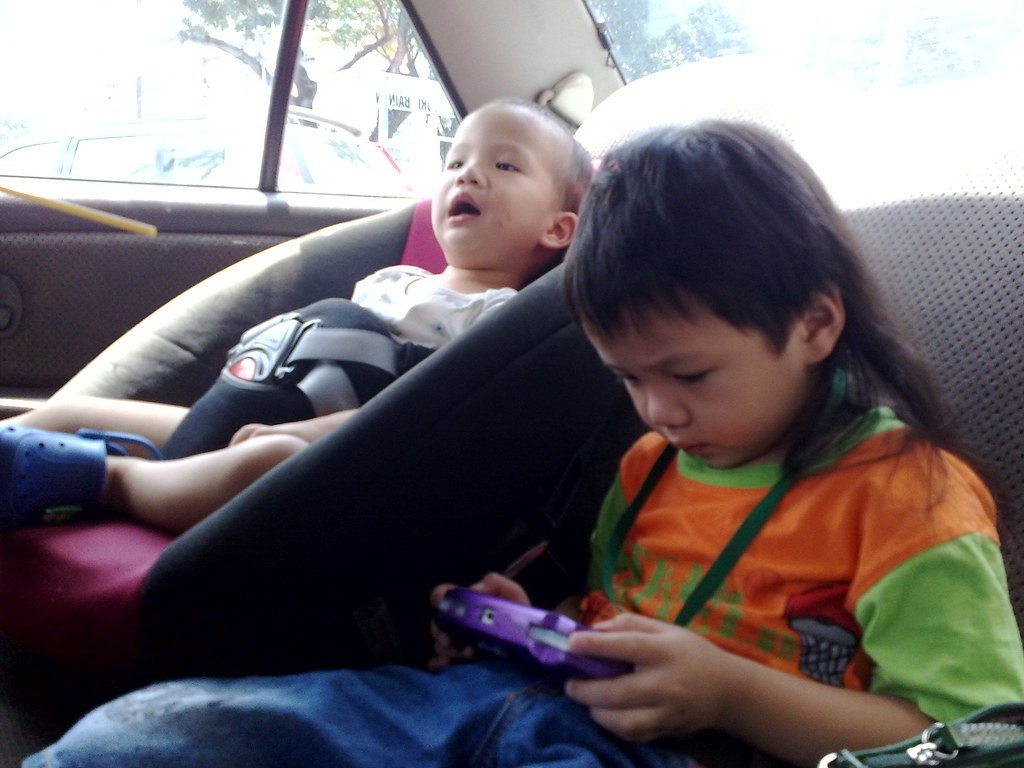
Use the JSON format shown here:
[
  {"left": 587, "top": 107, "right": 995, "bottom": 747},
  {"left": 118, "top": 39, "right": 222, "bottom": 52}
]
[{"left": 0, "top": 105, "right": 414, "bottom": 199}]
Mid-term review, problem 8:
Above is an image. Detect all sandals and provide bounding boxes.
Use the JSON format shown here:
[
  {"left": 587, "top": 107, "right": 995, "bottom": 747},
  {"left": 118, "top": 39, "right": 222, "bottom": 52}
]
[{"left": 0, "top": 426, "right": 161, "bottom": 521}]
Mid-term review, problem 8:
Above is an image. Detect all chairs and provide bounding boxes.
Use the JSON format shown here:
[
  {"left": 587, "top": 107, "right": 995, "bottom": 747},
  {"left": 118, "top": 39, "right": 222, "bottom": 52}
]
[
  {"left": 837, "top": 186, "right": 1024, "bottom": 648},
  {"left": 0, "top": 195, "right": 646, "bottom": 730}
]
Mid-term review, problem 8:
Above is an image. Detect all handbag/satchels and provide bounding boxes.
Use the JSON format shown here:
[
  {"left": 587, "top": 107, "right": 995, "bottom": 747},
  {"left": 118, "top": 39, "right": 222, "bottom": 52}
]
[{"left": 819, "top": 702, "right": 1024, "bottom": 768}]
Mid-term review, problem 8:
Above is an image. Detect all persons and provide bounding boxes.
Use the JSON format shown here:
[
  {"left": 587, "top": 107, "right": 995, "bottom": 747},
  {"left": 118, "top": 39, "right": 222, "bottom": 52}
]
[
  {"left": 0, "top": 100, "right": 593, "bottom": 528},
  {"left": 21, "top": 116, "right": 1024, "bottom": 767}
]
